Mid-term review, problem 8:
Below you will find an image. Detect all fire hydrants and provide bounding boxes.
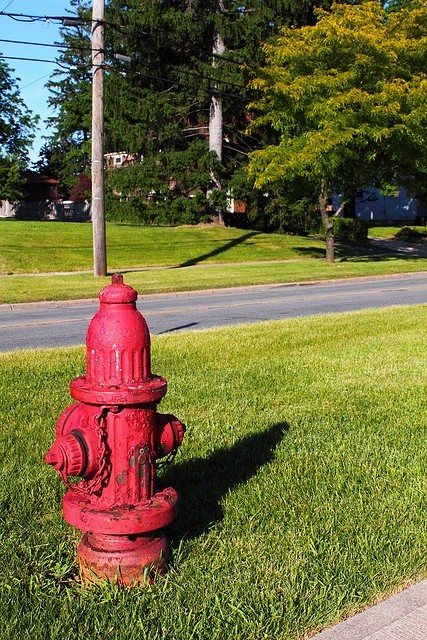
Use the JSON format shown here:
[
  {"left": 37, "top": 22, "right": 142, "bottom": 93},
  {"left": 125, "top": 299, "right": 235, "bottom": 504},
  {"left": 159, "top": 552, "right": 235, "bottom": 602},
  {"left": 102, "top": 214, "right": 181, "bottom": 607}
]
[{"left": 44, "top": 273, "right": 186, "bottom": 586}]
